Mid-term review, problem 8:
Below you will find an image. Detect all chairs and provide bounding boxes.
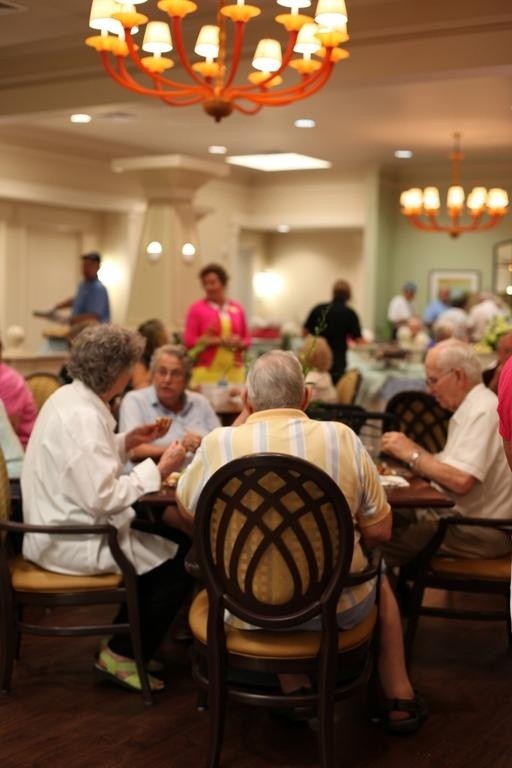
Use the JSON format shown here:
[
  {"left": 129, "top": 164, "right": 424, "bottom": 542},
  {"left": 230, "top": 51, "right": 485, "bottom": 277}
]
[
  {"left": 187, "top": 448, "right": 378, "bottom": 768},
  {"left": 0, "top": 316, "right": 510, "bottom": 461},
  {"left": 402, "top": 516, "right": 512, "bottom": 676},
  {"left": 2, "top": 487, "right": 162, "bottom": 711}
]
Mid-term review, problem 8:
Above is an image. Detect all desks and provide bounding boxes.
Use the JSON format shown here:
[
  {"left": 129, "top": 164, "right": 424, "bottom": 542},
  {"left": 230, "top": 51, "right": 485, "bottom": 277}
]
[{"left": 131, "top": 466, "right": 456, "bottom": 515}]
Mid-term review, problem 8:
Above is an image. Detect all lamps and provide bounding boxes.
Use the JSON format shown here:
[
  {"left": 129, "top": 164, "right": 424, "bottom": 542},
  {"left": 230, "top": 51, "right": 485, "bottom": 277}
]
[
  {"left": 396, "top": 126, "right": 511, "bottom": 244},
  {"left": 77, "top": 0, "right": 358, "bottom": 127}
]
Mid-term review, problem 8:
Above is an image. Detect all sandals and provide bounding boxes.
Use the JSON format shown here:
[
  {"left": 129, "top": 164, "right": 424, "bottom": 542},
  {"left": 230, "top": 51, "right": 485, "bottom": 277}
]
[
  {"left": 383, "top": 698, "right": 428, "bottom": 733},
  {"left": 286, "top": 687, "right": 318, "bottom": 718},
  {"left": 93, "top": 639, "right": 167, "bottom": 692}
]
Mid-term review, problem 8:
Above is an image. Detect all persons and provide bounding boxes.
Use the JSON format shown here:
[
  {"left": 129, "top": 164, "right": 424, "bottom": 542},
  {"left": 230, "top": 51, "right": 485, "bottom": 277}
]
[{"left": 1, "top": 253, "right": 512, "bottom": 737}]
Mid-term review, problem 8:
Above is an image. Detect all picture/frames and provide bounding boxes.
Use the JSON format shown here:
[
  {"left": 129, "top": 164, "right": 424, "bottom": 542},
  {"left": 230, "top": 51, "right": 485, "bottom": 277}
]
[
  {"left": 492, "top": 237, "right": 512, "bottom": 301},
  {"left": 423, "top": 267, "right": 483, "bottom": 307}
]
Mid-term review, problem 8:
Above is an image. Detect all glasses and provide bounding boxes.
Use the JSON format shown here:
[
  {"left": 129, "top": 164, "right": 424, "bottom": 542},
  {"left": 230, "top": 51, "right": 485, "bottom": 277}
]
[{"left": 426, "top": 370, "right": 454, "bottom": 388}]
